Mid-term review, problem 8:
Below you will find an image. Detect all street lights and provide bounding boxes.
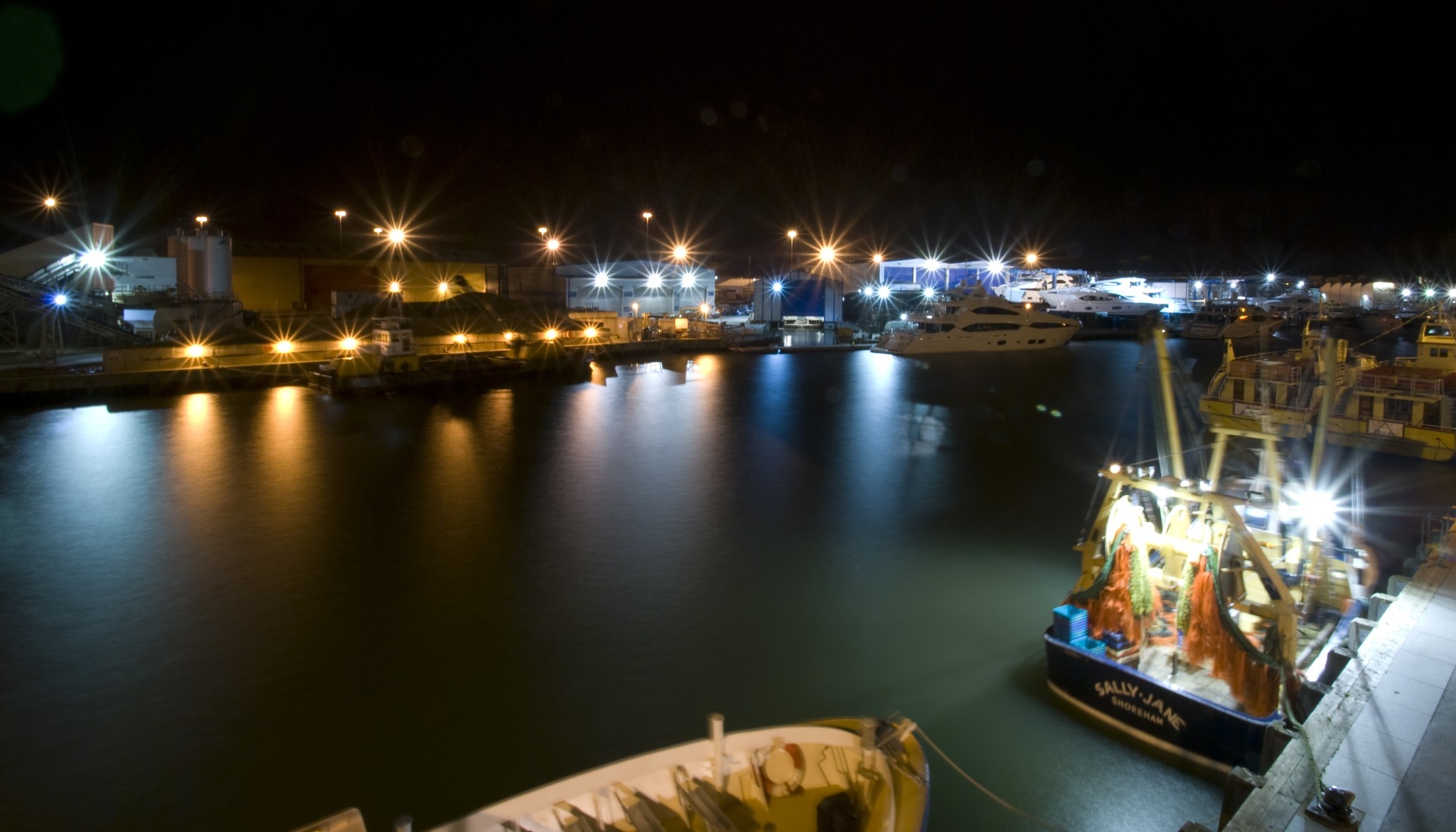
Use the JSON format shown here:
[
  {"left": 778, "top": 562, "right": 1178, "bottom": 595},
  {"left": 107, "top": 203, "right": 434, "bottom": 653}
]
[
  {"left": 534, "top": 223, "right": 559, "bottom": 318},
  {"left": 42, "top": 192, "right": 59, "bottom": 291},
  {"left": 592, "top": 206, "right": 805, "bottom": 329},
  {"left": 48, "top": 292, "right": 68, "bottom": 366},
  {"left": 814, "top": 229, "right": 1060, "bottom": 314},
  {"left": 387, "top": 224, "right": 407, "bottom": 324},
  {"left": 436, "top": 277, "right": 452, "bottom": 324},
  {"left": 337, "top": 206, "right": 347, "bottom": 244}
]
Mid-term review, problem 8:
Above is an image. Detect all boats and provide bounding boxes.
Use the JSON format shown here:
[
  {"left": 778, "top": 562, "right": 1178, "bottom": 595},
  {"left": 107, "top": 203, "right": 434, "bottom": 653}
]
[
  {"left": 870, "top": 276, "right": 1084, "bottom": 354},
  {"left": 1042, "top": 325, "right": 1379, "bottom": 793},
  {"left": 1163, "top": 291, "right": 1405, "bottom": 341},
  {"left": 281, "top": 707, "right": 938, "bottom": 827},
  {"left": 1197, "top": 318, "right": 1454, "bottom": 473},
  {"left": 994, "top": 273, "right": 1165, "bottom": 320}
]
